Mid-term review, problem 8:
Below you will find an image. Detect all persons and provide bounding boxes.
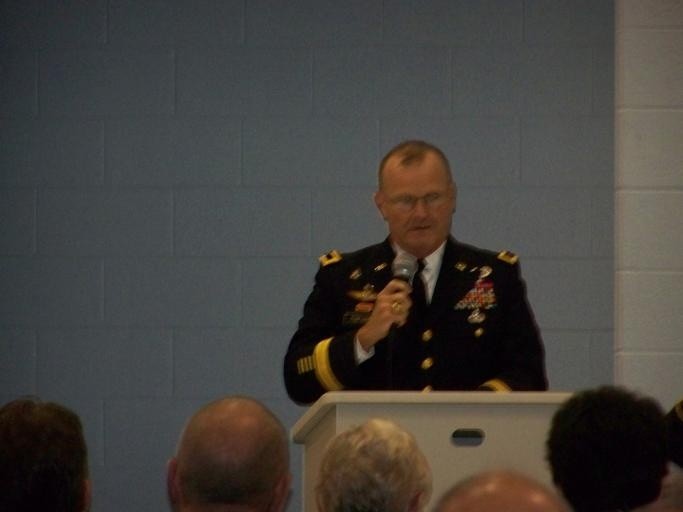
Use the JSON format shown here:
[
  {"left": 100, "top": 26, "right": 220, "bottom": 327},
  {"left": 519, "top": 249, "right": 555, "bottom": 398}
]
[
  {"left": 314, "top": 417, "right": 434, "bottom": 512},
  {"left": 282, "top": 141, "right": 549, "bottom": 404},
  {"left": 1, "top": 396, "right": 91, "bottom": 512},
  {"left": 548, "top": 386, "right": 670, "bottom": 512},
  {"left": 436, "top": 471, "right": 558, "bottom": 512},
  {"left": 167, "top": 397, "right": 290, "bottom": 512}
]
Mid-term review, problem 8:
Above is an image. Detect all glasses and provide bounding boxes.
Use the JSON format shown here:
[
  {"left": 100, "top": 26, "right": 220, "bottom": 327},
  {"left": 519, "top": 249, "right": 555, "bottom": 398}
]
[
  {"left": 381, "top": 183, "right": 452, "bottom": 212},
  {"left": 412, "top": 260, "right": 427, "bottom": 318}
]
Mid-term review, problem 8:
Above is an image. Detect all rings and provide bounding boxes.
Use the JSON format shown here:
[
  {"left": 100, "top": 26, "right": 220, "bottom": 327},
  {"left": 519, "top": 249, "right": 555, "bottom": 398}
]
[{"left": 392, "top": 303, "right": 402, "bottom": 314}]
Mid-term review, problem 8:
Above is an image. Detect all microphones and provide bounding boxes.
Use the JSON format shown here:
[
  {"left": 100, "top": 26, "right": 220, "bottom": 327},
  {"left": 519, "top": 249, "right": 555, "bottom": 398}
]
[{"left": 392, "top": 253, "right": 418, "bottom": 333}]
